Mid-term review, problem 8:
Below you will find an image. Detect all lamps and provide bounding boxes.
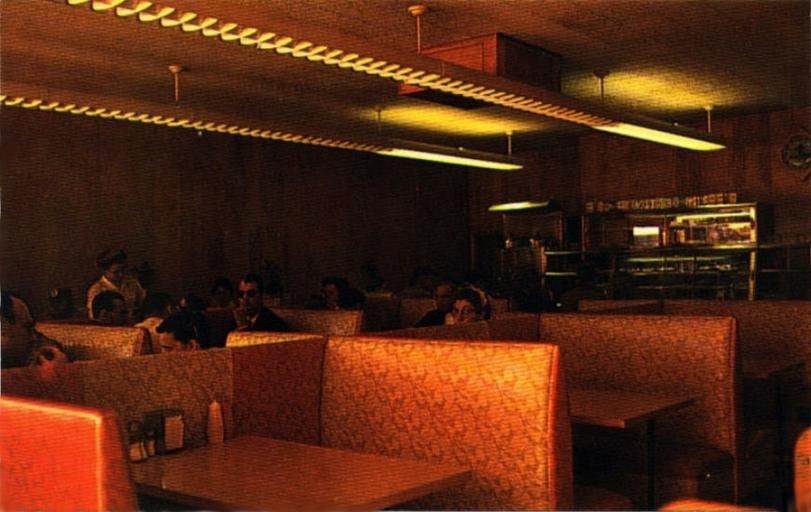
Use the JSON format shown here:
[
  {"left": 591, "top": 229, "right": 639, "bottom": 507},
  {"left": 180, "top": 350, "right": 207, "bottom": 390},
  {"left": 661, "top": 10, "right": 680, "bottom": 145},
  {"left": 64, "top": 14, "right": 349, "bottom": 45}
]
[
  {"left": 372, "top": 104, "right": 525, "bottom": 171},
  {"left": 588, "top": 67, "right": 727, "bottom": 151}
]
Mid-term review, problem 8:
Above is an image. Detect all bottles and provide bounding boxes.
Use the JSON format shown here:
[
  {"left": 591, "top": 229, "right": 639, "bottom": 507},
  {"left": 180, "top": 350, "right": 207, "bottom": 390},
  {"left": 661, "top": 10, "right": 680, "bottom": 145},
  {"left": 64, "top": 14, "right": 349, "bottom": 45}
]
[
  {"left": 207, "top": 399, "right": 224, "bottom": 444},
  {"left": 145, "top": 431, "right": 155, "bottom": 457}
]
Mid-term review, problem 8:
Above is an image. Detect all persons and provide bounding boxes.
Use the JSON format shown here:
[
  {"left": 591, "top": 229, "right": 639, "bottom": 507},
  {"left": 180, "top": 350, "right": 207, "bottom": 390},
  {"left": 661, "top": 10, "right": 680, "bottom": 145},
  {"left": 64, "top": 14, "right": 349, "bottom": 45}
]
[
  {"left": 413, "top": 279, "right": 489, "bottom": 329},
  {"left": 216, "top": 272, "right": 293, "bottom": 332},
  {"left": 88, "top": 250, "right": 145, "bottom": 327},
  {"left": 133, "top": 290, "right": 212, "bottom": 354},
  {"left": 314, "top": 276, "right": 388, "bottom": 313},
  {"left": 2, "top": 290, "right": 71, "bottom": 369}
]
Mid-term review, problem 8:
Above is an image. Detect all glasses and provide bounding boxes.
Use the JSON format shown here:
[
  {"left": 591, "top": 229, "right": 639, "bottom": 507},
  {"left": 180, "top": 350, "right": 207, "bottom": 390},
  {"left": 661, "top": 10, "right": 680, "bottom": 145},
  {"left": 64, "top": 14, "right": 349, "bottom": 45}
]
[{"left": 236, "top": 289, "right": 257, "bottom": 297}]
